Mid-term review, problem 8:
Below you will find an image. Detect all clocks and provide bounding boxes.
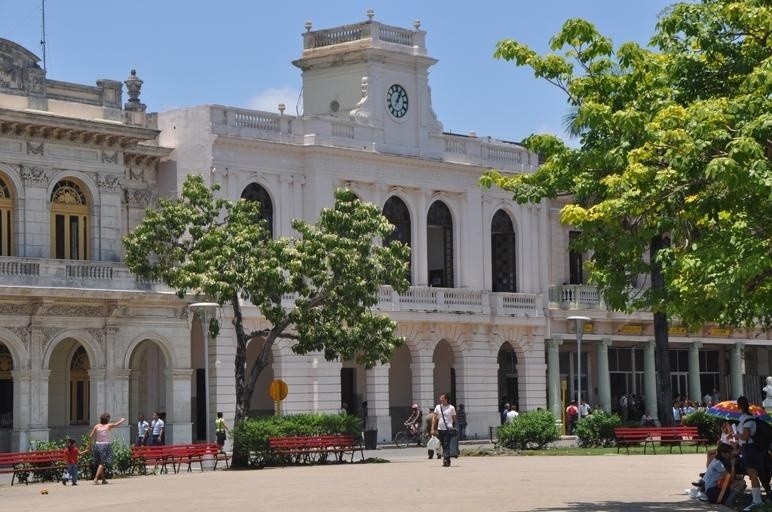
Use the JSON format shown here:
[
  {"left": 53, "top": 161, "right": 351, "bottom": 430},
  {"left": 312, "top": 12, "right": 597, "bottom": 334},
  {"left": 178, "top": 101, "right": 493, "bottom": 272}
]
[{"left": 387, "top": 84, "right": 408, "bottom": 118}]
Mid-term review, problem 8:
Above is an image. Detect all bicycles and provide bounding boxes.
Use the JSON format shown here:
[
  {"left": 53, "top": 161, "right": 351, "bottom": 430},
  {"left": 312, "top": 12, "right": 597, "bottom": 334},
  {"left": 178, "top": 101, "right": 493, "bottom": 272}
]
[{"left": 394, "top": 421, "right": 427, "bottom": 448}]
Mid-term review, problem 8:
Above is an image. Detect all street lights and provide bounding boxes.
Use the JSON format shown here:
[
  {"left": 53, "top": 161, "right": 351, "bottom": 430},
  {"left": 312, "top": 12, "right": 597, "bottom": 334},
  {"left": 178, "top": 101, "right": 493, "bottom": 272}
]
[{"left": 567, "top": 316, "right": 591, "bottom": 422}]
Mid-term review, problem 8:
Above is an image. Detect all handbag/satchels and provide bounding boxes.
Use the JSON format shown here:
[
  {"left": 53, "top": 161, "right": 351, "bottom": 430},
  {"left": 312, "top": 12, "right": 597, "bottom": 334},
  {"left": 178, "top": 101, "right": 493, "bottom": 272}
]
[
  {"left": 219, "top": 432, "right": 226, "bottom": 441},
  {"left": 447, "top": 428, "right": 457, "bottom": 436}
]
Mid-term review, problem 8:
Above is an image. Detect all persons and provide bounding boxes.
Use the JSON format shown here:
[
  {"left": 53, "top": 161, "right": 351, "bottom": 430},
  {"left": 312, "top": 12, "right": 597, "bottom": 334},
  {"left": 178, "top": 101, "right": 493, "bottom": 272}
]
[
  {"left": 619, "top": 390, "right": 720, "bottom": 435},
  {"left": 692, "top": 396, "right": 772, "bottom": 512},
  {"left": 135, "top": 411, "right": 165, "bottom": 447},
  {"left": 61, "top": 412, "right": 126, "bottom": 485},
  {"left": 501, "top": 402, "right": 520, "bottom": 425},
  {"left": 214, "top": 411, "right": 230, "bottom": 448},
  {"left": 404, "top": 392, "right": 467, "bottom": 466},
  {"left": 566, "top": 400, "right": 593, "bottom": 435}
]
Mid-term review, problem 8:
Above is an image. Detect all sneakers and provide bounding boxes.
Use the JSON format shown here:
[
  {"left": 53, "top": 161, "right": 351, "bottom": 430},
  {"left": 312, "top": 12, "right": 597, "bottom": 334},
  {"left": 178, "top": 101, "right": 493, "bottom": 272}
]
[
  {"left": 62, "top": 477, "right": 109, "bottom": 485},
  {"left": 444, "top": 459, "right": 450, "bottom": 466},
  {"left": 428, "top": 453, "right": 441, "bottom": 459},
  {"left": 743, "top": 501, "right": 765, "bottom": 511}
]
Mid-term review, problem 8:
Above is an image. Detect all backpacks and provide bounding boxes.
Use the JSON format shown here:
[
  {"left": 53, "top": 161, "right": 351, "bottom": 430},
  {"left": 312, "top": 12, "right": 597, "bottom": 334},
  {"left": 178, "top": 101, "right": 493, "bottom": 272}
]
[{"left": 744, "top": 417, "right": 772, "bottom": 449}]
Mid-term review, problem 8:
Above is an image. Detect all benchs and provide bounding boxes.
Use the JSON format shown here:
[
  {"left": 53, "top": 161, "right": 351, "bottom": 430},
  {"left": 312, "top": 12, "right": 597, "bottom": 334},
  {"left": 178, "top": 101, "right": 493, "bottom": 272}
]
[
  {"left": 614, "top": 426, "right": 709, "bottom": 454},
  {"left": 128, "top": 442, "right": 235, "bottom": 475},
  {"left": 268, "top": 434, "right": 365, "bottom": 465},
  {"left": 1, "top": 448, "right": 93, "bottom": 485}
]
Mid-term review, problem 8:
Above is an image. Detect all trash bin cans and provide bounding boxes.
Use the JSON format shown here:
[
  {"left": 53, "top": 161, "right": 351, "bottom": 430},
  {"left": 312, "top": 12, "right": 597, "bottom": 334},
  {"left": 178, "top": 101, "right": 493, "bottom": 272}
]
[
  {"left": 94, "top": 445, "right": 113, "bottom": 479},
  {"left": 365, "top": 430, "right": 377, "bottom": 450}
]
